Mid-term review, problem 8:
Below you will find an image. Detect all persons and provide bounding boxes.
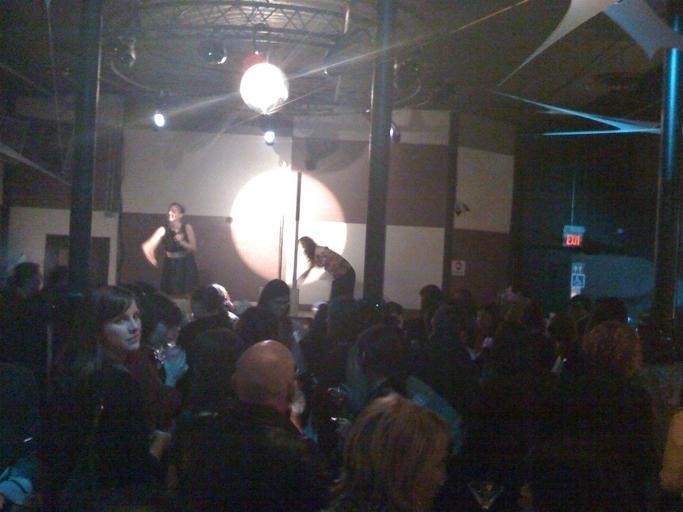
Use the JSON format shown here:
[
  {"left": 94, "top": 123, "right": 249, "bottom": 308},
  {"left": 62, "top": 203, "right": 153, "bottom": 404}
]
[
  {"left": 139, "top": 200, "right": 203, "bottom": 298},
  {"left": 290, "top": 236, "right": 358, "bottom": 307},
  {"left": 1, "top": 262, "right": 682, "bottom": 512}
]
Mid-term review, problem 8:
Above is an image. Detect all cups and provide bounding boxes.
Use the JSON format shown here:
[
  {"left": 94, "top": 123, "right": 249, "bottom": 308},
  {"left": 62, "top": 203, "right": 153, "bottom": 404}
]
[{"left": 163, "top": 342, "right": 181, "bottom": 363}]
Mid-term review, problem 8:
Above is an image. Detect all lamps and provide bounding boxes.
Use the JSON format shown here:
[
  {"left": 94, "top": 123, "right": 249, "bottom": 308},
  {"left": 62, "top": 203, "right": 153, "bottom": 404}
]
[
  {"left": 236, "top": 52, "right": 290, "bottom": 116},
  {"left": 106, "top": 28, "right": 145, "bottom": 68},
  {"left": 195, "top": 27, "right": 228, "bottom": 66}
]
